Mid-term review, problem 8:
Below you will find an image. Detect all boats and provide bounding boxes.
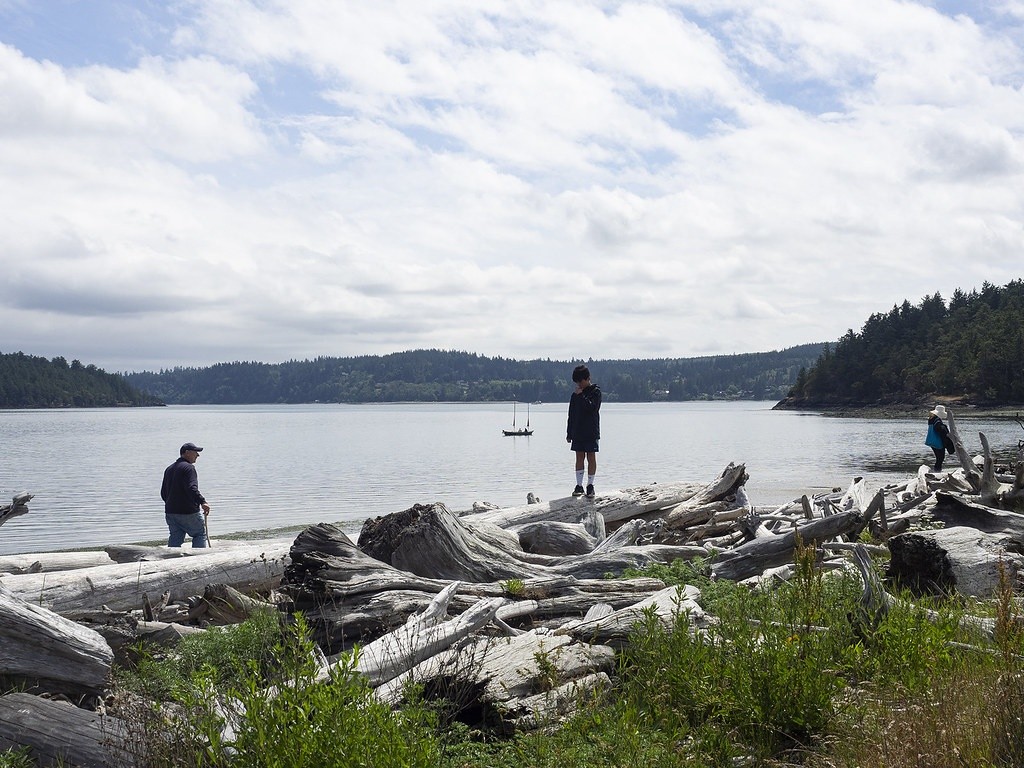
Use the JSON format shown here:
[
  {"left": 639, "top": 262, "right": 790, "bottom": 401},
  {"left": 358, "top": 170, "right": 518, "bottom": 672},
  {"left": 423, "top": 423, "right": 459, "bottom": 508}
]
[
  {"left": 502, "top": 402, "right": 534, "bottom": 436},
  {"left": 0, "top": 492, "right": 34, "bottom": 525}
]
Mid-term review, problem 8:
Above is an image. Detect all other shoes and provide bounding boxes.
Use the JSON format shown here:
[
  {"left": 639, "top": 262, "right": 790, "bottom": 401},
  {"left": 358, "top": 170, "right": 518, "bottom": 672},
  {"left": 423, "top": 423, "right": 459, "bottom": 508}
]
[
  {"left": 586, "top": 484, "right": 596, "bottom": 497},
  {"left": 572, "top": 485, "right": 585, "bottom": 495}
]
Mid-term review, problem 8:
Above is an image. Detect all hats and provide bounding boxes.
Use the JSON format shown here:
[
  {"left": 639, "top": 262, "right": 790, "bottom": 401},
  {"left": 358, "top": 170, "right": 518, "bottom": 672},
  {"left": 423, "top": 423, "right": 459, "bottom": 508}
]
[
  {"left": 180, "top": 443, "right": 203, "bottom": 452},
  {"left": 930, "top": 405, "right": 947, "bottom": 419}
]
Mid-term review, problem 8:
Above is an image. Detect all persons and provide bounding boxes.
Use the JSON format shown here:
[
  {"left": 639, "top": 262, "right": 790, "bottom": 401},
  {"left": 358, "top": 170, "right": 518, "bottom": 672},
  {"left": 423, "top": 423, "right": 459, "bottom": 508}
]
[
  {"left": 160, "top": 442, "right": 210, "bottom": 548},
  {"left": 928, "top": 405, "right": 950, "bottom": 473},
  {"left": 566, "top": 365, "right": 602, "bottom": 496}
]
